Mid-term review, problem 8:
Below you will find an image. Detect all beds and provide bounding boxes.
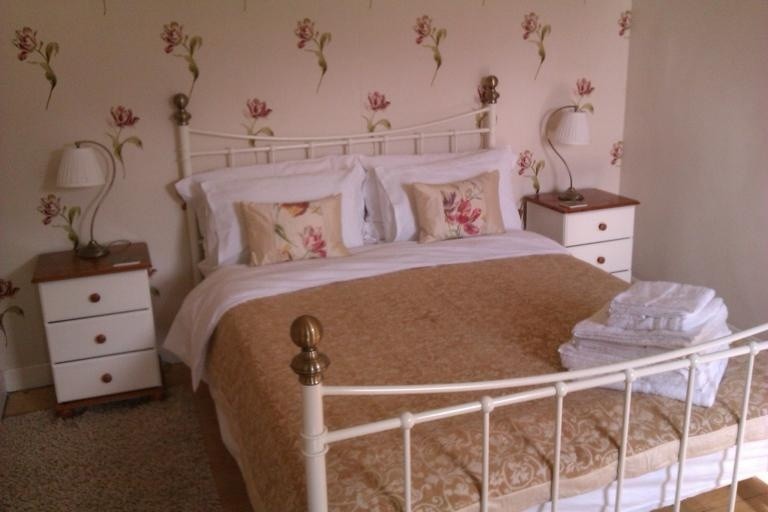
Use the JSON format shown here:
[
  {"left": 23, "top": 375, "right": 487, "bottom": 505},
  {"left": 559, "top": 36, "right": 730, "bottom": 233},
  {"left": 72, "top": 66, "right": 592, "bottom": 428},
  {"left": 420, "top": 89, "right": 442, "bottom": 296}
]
[{"left": 153, "top": 75, "right": 767, "bottom": 512}]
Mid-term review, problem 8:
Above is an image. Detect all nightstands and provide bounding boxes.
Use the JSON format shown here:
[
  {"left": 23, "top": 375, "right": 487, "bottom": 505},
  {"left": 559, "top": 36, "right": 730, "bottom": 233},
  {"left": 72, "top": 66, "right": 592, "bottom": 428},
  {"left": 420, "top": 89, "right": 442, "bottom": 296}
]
[
  {"left": 520, "top": 186, "right": 640, "bottom": 285},
  {"left": 28, "top": 241, "right": 175, "bottom": 421}
]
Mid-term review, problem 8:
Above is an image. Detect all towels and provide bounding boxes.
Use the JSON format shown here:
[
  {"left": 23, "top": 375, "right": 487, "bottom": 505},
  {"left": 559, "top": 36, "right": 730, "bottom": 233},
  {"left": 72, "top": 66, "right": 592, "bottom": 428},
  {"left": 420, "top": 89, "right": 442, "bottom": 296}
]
[
  {"left": 238, "top": 192, "right": 352, "bottom": 267},
  {"left": 557, "top": 277, "right": 735, "bottom": 409}
]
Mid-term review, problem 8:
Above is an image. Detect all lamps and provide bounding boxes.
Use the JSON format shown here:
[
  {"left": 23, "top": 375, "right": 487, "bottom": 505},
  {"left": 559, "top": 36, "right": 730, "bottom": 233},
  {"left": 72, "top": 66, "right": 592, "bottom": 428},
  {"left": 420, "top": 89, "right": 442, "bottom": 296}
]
[
  {"left": 542, "top": 104, "right": 592, "bottom": 201},
  {"left": 52, "top": 138, "right": 116, "bottom": 260}
]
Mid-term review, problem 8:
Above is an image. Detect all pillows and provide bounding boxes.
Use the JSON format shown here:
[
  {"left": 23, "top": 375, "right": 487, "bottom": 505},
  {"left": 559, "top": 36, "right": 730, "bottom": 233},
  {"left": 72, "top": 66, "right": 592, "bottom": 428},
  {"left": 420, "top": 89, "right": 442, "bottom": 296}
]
[
  {"left": 372, "top": 145, "right": 522, "bottom": 244},
  {"left": 409, "top": 167, "right": 507, "bottom": 246},
  {"left": 172, "top": 154, "right": 378, "bottom": 274},
  {"left": 355, "top": 144, "right": 513, "bottom": 245},
  {"left": 196, "top": 157, "right": 366, "bottom": 269}
]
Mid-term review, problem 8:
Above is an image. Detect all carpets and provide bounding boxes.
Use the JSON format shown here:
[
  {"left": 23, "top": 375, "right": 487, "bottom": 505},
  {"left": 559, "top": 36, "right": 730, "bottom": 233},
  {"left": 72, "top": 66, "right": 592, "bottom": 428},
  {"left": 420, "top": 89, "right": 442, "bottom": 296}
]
[{"left": 0, "top": 377, "right": 225, "bottom": 512}]
